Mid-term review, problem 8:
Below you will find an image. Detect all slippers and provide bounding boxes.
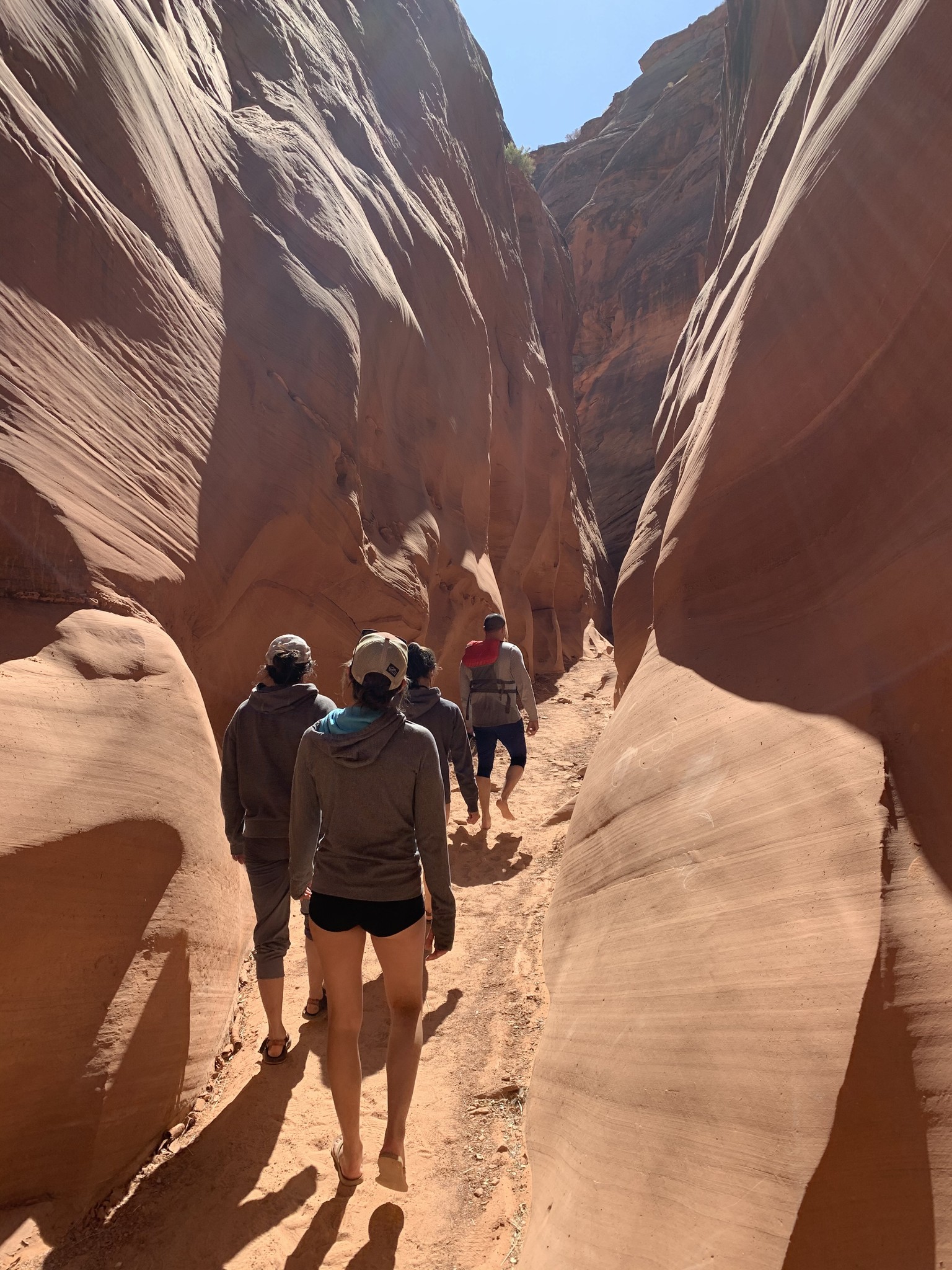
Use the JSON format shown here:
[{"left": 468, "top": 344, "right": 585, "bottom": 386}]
[
  {"left": 331, "top": 1135, "right": 364, "bottom": 1186},
  {"left": 375, "top": 1152, "right": 409, "bottom": 1192}
]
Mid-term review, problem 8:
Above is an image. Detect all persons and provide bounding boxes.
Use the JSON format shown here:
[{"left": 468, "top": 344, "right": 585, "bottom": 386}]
[
  {"left": 218, "top": 635, "right": 338, "bottom": 1065},
  {"left": 459, "top": 612, "right": 538, "bottom": 830},
  {"left": 401, "top": 640, "right": 481, "bottom": 924},
  {"left": 288, "top": 630, "right": 459, "bottom": 1194}
]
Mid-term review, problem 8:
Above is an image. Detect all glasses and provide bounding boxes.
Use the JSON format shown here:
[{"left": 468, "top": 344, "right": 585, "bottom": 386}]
[{"left": 351, "top": 629, "right": 407, "bottom": 669}]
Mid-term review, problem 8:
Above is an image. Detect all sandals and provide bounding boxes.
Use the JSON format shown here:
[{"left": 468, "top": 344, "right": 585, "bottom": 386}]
[
  {"left": 302, "top": 988, "right": 328, "bottom": 1018},
  {"left": 261, "top": 1029, "right": 294, "bottom": 1064}
]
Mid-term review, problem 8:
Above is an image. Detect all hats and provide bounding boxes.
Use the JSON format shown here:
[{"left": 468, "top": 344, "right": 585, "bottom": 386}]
[
  {"left": 265, "top": 633, "right": 311, "bottom": 665},
  {"left": 352, "top": 632, "right": 408, "bottom": 691}
]
